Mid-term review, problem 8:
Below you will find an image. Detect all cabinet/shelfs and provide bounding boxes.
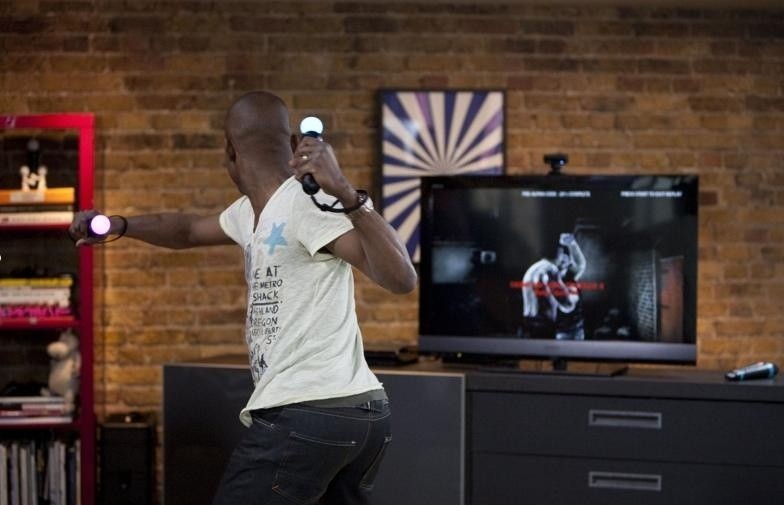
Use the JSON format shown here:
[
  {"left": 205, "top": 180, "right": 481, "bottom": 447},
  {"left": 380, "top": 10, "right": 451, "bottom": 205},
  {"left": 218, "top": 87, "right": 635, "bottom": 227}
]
[{"left": 1, "top": 111, "right": 95, "bottom": 504}]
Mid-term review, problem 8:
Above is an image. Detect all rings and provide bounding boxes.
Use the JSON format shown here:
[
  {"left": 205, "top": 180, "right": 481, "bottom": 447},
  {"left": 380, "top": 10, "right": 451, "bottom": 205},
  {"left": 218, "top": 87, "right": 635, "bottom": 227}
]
[{"left": 301, "top": 155, "right": 307, "bottom": 163}]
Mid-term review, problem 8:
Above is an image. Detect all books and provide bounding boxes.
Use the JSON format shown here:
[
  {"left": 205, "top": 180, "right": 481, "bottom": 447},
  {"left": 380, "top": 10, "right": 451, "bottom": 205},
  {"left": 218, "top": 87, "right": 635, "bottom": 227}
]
[
  {"left": 0, "top": 187, "right": 76, "bottom": 231},
  {"left": 0, "top": 278, "right": 76, "bottom": 329},
  {"left": 0, "top": 438, "right": 82, "bottom": 505},
  {"left": 0, "top": 395, "right": 74, "bottom": 425}
]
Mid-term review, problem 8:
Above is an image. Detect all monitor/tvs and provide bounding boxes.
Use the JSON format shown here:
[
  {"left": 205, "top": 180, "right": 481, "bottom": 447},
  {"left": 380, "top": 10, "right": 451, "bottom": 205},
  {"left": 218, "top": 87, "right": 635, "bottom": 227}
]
[{"left": 417, "top": 173, "right": 700, "bottom": 378}]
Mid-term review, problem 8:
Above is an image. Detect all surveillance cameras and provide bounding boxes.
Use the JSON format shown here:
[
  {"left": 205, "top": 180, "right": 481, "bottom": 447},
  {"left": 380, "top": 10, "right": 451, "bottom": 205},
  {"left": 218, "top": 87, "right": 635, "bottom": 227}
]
[{"left": 543, "top": 153, "right": 568, "bottom": 173}]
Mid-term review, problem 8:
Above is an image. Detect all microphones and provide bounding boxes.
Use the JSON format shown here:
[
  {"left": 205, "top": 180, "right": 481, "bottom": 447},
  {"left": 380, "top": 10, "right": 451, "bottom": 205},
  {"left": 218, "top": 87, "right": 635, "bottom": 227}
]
[{"left": 724, "top": 360, "right": 778, "bottom": 382}]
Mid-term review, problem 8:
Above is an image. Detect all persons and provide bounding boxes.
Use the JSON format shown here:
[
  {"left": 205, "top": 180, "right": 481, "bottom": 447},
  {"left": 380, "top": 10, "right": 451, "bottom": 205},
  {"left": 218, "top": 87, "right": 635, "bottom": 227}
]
[
  {"left": 541, "top": 233, "right": 587, "bottom": 340},
  {"left": 71, "top": 92, "right": 417, "bottom": 505}
]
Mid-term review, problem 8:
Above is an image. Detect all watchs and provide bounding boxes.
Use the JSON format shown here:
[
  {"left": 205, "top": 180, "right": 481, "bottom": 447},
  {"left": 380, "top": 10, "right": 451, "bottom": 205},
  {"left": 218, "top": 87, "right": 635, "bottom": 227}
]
[{"left": 346, "top": 193, "right": 374, "bottom": 220}]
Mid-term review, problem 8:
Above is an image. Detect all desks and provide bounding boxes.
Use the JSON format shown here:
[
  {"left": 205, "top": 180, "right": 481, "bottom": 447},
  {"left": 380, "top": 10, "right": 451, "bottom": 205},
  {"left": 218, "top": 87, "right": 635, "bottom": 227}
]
[
  {"left": 466, "top": 365, "right": 783, "bottom": 504},
  {"left": 162, "top": 354, "right": 486, "bottom": 504}
]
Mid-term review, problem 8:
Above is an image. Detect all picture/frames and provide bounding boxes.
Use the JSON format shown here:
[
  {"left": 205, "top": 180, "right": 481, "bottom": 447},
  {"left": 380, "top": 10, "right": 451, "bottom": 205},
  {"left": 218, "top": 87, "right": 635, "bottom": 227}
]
[{"left": 376, "top": 87, "right": 507, "bottom": 269}]
